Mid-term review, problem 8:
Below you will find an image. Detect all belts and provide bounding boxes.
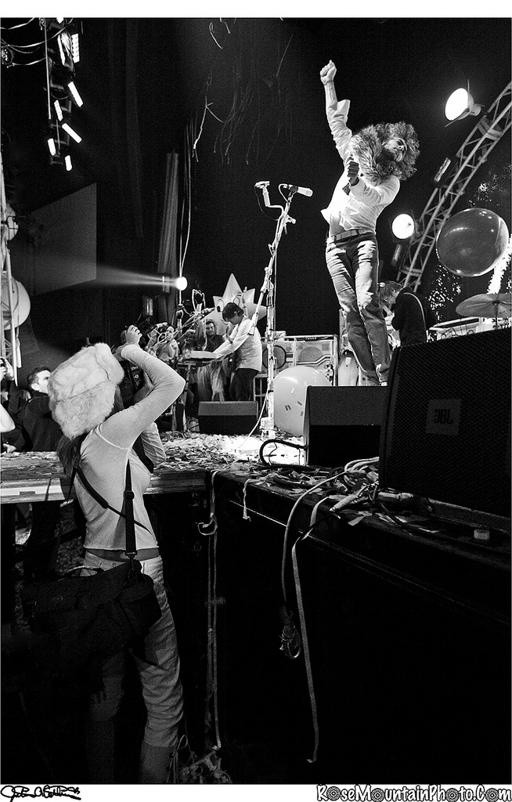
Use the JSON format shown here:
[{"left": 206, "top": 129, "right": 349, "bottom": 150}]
[{"left": 326, "top": 229, "right": 379, "bottom": 245}]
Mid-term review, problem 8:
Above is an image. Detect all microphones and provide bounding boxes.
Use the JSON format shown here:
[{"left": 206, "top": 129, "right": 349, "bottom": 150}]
[
  {"left": 201, "top": 308, "right": 215, "bottom": 319},
  {"left": 284, "top": 185, "right": 313, "bottom": 197}
]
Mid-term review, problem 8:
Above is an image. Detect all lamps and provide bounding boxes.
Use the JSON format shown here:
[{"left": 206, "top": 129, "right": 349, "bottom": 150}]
[
  {"left": 391, "top": 211, "right": 420, "bottom": 242},
  {"left": 445, "top": 78, "right": 481, "bottom": 127}
]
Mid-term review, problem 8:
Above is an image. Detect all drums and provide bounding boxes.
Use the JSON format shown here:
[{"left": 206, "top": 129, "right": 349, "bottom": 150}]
[{"left": 428, "top": 317, "right": 485, "bottom": 340}]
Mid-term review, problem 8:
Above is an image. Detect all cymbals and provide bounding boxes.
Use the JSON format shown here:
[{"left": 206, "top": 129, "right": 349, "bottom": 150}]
[{"left": 455, "top": 292, "right": 512, "bottom": 319}]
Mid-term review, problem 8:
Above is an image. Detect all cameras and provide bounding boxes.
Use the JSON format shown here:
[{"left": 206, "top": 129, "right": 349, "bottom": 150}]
[
  {"left": 122, "top": 320, "right": 157, "bottom": 347},
  {"left": 0, "top": 358, "right": 5, "bottom": 367}
]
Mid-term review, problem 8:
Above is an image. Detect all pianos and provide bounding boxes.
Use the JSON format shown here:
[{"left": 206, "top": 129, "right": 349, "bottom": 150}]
[{"left": 176, "top": 350, "right": 222, "bottom": 370}]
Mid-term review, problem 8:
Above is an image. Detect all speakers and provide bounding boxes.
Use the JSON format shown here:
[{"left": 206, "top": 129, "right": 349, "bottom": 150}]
[
  {"left": 303, "top": 386, "right": 385, "bottom": 465},
  {"left": 198, "top": 401, "right": 260, "bottom": 435},
  {"left": 261, "top": 336, "right": 294, "bottom": 375},
  {"left": 379, "top": 327, "right": 511, "bottom": 518},
  {"left": 293, "top": 334, "right": 339, "bottom": 386},
  {"left": 266, "top": 365, "right": 333, "bottom": 437}
]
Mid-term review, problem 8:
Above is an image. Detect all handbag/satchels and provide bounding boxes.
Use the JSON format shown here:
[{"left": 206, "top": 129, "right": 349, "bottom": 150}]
[{"left": 36, "top": 560, "right": 159, "bottom": 674}]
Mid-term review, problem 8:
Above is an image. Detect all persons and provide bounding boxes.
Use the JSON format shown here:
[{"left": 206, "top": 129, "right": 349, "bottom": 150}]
[
  {"left": 46, "top": 325, "right": 188, "bottom": 784},
  {"left": 114, "top": 302, "right": 262, "bottom": 435},
  {"left": 317, "top": 58, "right": 421, "bottom": 388},
  {"left": 0, "top": 356, "right": 63, "bottom": 453}
]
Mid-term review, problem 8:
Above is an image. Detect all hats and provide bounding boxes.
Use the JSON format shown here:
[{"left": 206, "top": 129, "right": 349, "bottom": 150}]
[{"left": 48, "top": 342, "right": 124, "bottom": 441}]
